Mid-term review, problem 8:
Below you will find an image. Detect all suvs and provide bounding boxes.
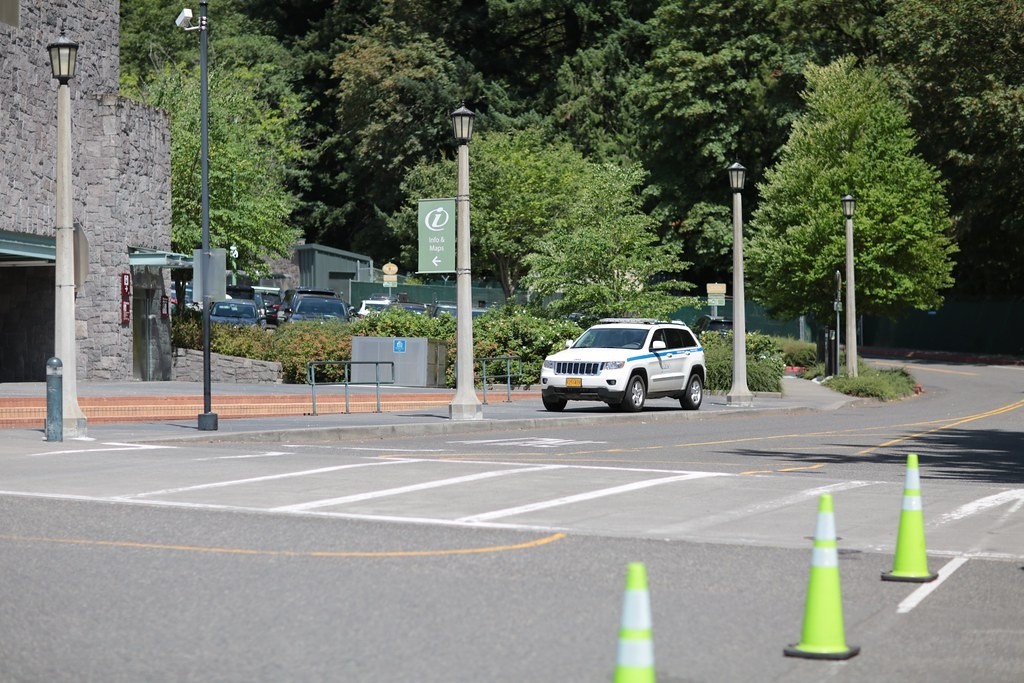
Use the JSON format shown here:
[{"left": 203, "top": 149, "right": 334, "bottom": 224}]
[{"left": 537, "top": 316, "right": 704, "bottom": 414}]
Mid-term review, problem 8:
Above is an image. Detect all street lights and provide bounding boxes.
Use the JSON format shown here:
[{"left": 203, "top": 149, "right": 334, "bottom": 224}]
[
  {"left": 840, "top": 191, "right": 861, "bottom": 378},
  {"left": 450, "top": 99, "right": 480, "bottom": 421},
  {"left": 42, "top": 15, "right": 88, "bottom": 438},
  {"left": 727, "top": 158, "right": 751, "bottom": 403}
]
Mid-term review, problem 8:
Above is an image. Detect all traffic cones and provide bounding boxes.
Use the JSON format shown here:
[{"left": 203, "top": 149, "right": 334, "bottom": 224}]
[
  {"left": 780, "top": 490, "right": 861, "bottom": 660},
  {"left": 879, "top": 451, "right": 939, "bottom": 582},
  {"left": 611, "top": 561, "right": 658, "bottom": 682}
]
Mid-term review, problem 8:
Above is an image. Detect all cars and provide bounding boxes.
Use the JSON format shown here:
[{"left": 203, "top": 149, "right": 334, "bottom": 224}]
[{"left": 168, "top": 279, "right": 489, "bottom": 333}]
